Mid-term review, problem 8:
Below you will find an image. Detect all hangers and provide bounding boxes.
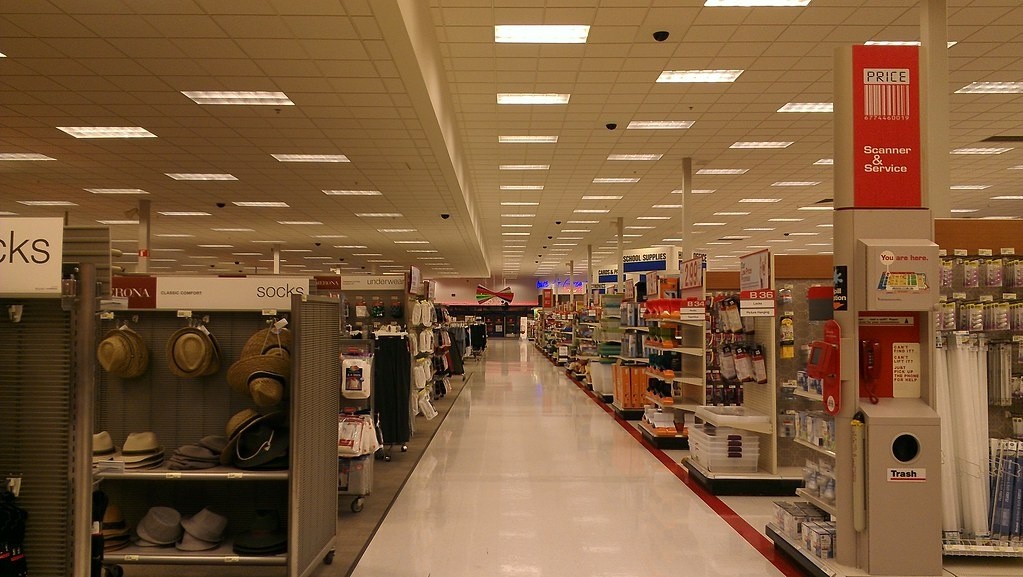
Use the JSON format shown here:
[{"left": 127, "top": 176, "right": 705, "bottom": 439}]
[
  {"left": 640, "top": 298, "right": 696, "bottom": 437},
  {"left": 452, "top": 317, "right": 485, "bottom": 328}
]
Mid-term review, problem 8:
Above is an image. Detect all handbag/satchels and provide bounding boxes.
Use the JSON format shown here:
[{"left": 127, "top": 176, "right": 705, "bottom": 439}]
[
  {"left": 338, "top": 411, "right": 380, "bottom": 459},
  {"left": 339, "top": 352, "right": 376, "bottom": 400}
]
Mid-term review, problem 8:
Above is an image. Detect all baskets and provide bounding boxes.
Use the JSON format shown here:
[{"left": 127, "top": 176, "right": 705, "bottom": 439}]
[{"left": 687, "top": 422, "right": 760, "bottom": 474}]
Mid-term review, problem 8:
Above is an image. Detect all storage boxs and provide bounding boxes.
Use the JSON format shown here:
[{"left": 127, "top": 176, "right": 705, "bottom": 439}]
[
  {"left": 611, "top": 277, "right": 679, "bottom": 410},
  {"left": 589, "top": 360, "right": 617, "bottom": 394},
  {"left": 600, "top": 294, "right": 622, "bottom": 315},
  {"left": 801, "top": 449, "right": 836, "bottom": 506},
  {"left": 600, "top": 318, "right": 624, "bottom": 339},
  {"left": 339, "top": 457, "right": 371, "bottom": 494},
  {"left": 686, "top": 422, "right": 761, "bottom": 476},
  {"left": 715, "top": 299, "right": 768, "bottom": 385},
  {"left": 796, "top": 371, "right": 824, "bottom": 396},
  {"left": 793, "top": 409, "right": 835, "bottom": 452},
  {"left": 771, "top": 498, "right": 836, "bottom": 559}
]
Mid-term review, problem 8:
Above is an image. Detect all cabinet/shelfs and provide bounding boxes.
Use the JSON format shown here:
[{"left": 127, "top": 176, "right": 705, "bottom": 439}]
[
  {"left": 587, "top": 315, "right": 622, "bottom": 402},
  {"left": 766, "top": 386, "right": 868, "bottom": 577},
  {"left": 637, "top": 262, "right": 746, "bottom": 450},
  {"left": 340, "top": 272, "right": 436, "bottom": 513},
  {"left": 680, "top": 248, "right": 835, "bottom": 496},
  {"left": 528, "top": 300, "right": 600, "bottom": 392},
  {"left": 0, "top": 216, "right": 339, "bottom": 576}
]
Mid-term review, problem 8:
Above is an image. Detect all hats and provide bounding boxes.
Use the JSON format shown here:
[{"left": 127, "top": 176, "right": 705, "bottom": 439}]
[
  {"left": 93, "top": 318, "right": 291, "bottom": 471},
  {"left": 100, "top": 502, "right": 288, "bottom": 557}
]
